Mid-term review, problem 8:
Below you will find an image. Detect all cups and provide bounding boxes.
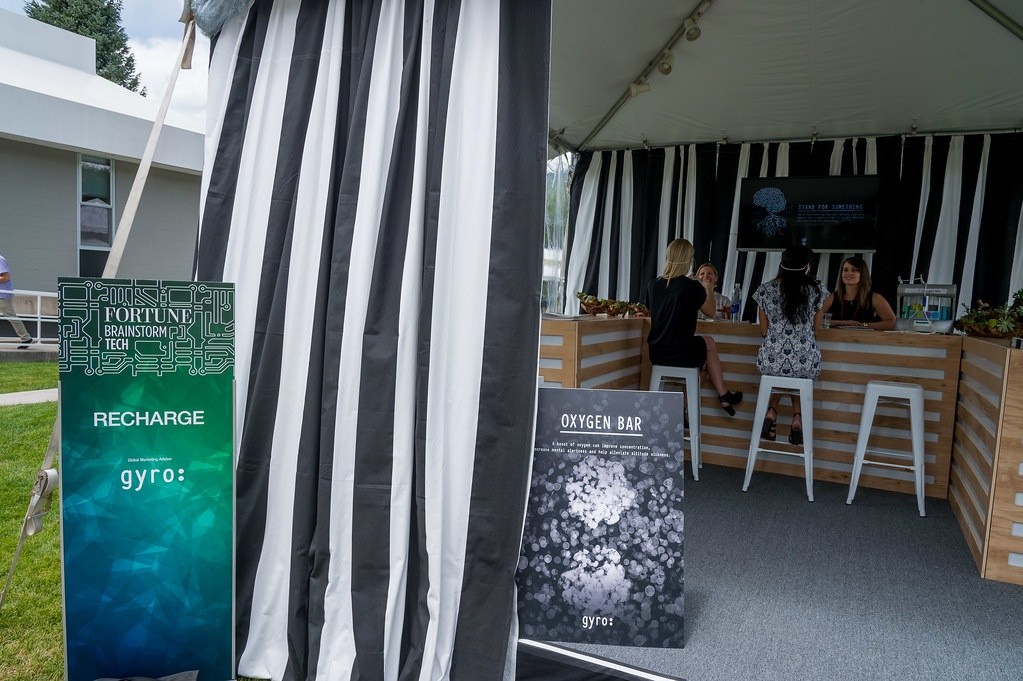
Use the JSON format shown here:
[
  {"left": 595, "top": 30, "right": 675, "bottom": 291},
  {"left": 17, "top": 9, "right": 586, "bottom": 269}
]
[{"left": 819, "top": 312, "right": 833, "bottom": 329}]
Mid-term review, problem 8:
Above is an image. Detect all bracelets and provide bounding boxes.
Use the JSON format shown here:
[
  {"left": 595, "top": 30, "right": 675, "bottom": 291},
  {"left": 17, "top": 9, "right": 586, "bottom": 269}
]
[{"left": 863, "top": 322, "right": 871, "bottom": 327}]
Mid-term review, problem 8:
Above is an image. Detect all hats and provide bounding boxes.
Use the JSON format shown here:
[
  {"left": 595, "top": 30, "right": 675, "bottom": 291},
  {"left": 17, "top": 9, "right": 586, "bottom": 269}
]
[{"left": 779, "top": 244, "right": 814, "bottom": 276}]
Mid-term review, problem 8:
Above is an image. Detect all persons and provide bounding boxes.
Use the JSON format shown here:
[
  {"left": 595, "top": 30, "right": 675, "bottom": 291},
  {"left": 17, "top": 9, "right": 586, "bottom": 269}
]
[
  {"left": 0, "top": 255, "right": 33, "bottom": 350},
  {"left": 644, "top": 239, "right": 743, "bottom": 416},
  {"left": 820, "top": 256, "right": 897, "bottom": 331},
  {"left": 691, "top": 262, "right": 732, "bottom": 322},
  {"left": 751, "top": 244, "right": 823, "bottom": 445}
]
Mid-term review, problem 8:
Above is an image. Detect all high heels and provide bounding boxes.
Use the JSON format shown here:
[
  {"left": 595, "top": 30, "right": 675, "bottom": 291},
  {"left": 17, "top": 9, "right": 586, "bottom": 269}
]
[
  {"left": 718, "top": 390, "right": 744, "bottom": 416},
  {"left": 760, "top": 409, "right": 777, "bottom": 441},
  {"left": 787, "top": 413, "right": 803, "bottom": 445}
]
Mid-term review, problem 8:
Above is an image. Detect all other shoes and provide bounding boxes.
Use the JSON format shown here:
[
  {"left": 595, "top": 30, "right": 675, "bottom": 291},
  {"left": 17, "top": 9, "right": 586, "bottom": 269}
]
[{"left": 17, "top": 339, "right": 33, "bottom": 349}]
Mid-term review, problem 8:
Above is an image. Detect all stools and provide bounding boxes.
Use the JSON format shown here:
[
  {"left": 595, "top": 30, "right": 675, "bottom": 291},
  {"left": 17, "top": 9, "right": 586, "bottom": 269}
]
[
  {"left": 743, "top": 374, "right": 815, "bottom": 501},
  {"left": 846, "top": 380, "right": 925, "bottom": 517},
  {"left": 650, "top": 366, "right": 702, "bottom": 480}
]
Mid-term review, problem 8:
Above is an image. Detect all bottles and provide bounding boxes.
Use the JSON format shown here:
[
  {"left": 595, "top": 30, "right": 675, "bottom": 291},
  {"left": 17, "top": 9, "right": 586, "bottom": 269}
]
[
  {"left": 730, "top": 283, "right": 742, "bottom": 324},
  {"left": 902, "top": 296, "right": 951, "bottom": 320}
]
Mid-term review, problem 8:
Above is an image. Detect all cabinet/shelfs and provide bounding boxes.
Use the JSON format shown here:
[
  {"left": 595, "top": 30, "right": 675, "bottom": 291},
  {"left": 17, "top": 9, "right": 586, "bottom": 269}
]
[{"left": 538, "top": 315, "right": 1023, "bottom": 585}]
